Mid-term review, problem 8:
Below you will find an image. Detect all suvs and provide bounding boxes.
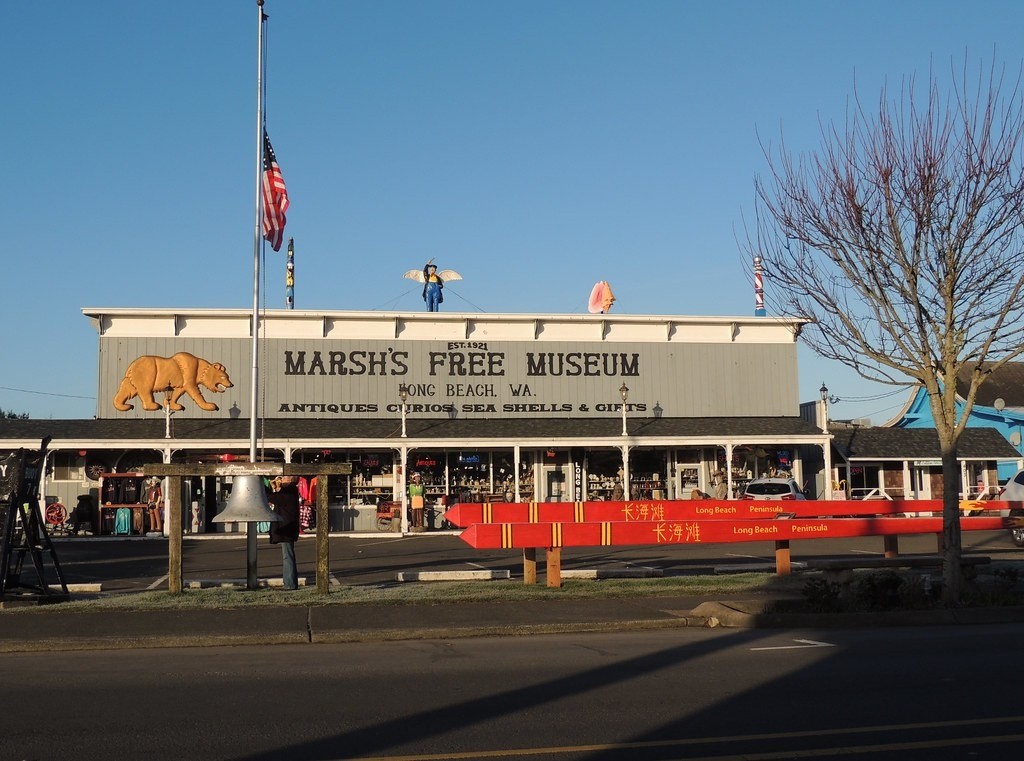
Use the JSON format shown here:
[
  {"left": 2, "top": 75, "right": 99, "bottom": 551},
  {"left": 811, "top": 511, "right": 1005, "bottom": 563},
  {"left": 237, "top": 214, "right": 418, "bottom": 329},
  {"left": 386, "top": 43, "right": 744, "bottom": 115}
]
[
  {"left": 743, "top": 477, "right": 807, "bottom": 509},
  {"left": 1000, "top": 467, "right": 1024, "bottom": 547}
]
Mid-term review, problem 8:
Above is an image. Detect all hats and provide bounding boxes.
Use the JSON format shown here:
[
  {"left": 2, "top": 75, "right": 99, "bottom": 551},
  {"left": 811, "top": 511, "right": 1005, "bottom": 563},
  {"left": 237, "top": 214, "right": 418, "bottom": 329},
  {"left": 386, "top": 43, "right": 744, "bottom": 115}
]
[
  {"left": 412, "top": 472, "right": 421, "bottom": 478},
  {"left": 148, "top": 476, "right": 161, "bottom": 482}
]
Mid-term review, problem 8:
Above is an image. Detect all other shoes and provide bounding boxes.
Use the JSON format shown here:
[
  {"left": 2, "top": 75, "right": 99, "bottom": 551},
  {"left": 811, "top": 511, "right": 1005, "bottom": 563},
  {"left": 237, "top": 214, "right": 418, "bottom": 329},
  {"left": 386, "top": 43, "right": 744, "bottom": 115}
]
[{"left": 278, "top": 588, "right": 296, "bottom": 590}]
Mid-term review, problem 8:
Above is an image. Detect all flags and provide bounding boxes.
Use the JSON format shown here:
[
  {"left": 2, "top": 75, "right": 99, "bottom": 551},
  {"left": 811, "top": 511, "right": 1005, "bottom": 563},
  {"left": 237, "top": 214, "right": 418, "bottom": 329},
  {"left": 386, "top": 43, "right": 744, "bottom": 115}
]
[{"left": 261, "top": 127, "right": 291, "bottom": 251}]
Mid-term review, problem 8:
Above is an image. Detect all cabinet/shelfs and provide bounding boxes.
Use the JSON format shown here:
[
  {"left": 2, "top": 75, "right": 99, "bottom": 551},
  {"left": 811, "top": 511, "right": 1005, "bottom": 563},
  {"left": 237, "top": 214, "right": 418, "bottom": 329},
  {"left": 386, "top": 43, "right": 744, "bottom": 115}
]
[{"left": 98, "top": 472, "right": 166, "bottom": 535}]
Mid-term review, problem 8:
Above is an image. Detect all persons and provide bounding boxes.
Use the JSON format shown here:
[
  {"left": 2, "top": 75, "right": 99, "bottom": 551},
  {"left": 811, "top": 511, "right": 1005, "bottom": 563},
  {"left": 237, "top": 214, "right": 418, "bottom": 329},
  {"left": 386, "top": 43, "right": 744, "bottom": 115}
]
[
  {"left": 422, "top": 257, "right": 443, "bottom": 312},
  {"left": 971, "top": 479, "right": 986, "bottom": 514},
  {"left": 711, "top": 471, "right": 728, "bottom": 500},
  {"left": 147, "top": 477, "right": 162, "bottom": 531},
  {"left": 409, "top": 472, "right": 426, "bottom": 528},
  {"left": 270, "top": 476, "right": 282, "bottom": 493},
  {"left": 264, "top": 476, "right": 300, "bottom": 590}
]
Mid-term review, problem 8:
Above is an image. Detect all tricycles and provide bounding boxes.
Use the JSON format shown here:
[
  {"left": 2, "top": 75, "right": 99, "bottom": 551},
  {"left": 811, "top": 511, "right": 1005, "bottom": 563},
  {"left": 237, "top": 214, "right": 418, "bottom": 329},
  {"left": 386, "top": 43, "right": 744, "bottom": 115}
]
[{"left": 377, "top": 499, "right": 440, "bottom": 530}]
